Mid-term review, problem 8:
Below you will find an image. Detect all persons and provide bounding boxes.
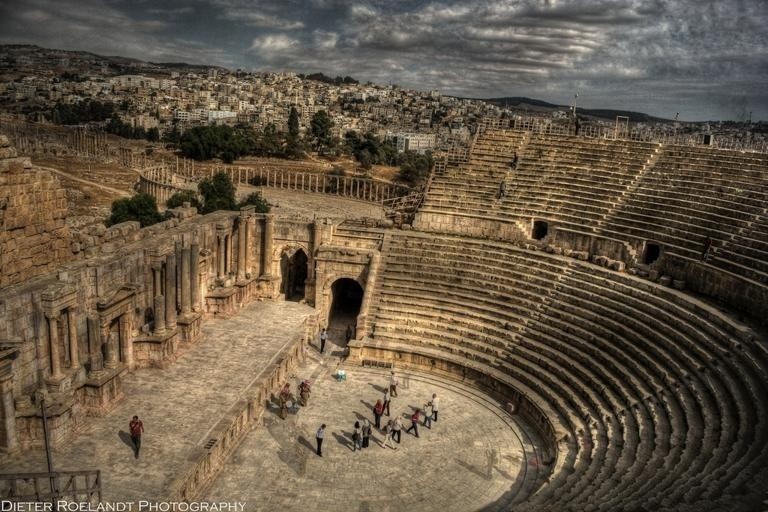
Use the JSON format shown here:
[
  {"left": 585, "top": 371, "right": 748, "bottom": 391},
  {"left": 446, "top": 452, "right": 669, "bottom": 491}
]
[
  {"left": 280, "top": 379, "right": 312, "bottom": 420},
  {"left": 130, "top": 415, "right": 144, "bottom": 459},
  {"left": 374, "top": 399, "right": 383, "bottom": 429},
  {"left": 382, "top": 388, "right": 391, "bottom": 416},
  {"left": 352, "top": 421, "right": 362, "bottom": 452},
  {"left": 499, "top": 178, "right": 506, "bottom": 199},
  {"left": 390, "top": 371, "right": 399, "bottom": 396},
  {"left": 338, "top": 368, "right": 345, "bottom": 382},
  {"left": 511, "top": 152, "right": 519, "bottom": 172},
  {"left": 343, "top": 347, "right": 349, "bottom": 357},
  {"left": 423, "top": 402, "right": 432, "bottom": 429},
  {"left": 431, "top": 394, "right": 439, "bottom": 421},
  {"left": 320, "top": 328, "right": 326, "bottom": 354},
  {"left": 346, "top": 325, "right": 352, "bottom": 344},
  {"left": 362, "top": 418, "right": 371, "bottom": 448},
  {"left": 406, "top": 410, "right": 421, "bottom": 438},
  {"left": 392, "top": 416, "right": 404, "bottom": 443},
  {"left": 382, "top": 420, "right": 397, "bottom": 449},
  {"left": 700, "top": 234, "right": 712, "bottom": 262},
  {"left": 316, "top": 424, "right": 326, "bottom": 456}
]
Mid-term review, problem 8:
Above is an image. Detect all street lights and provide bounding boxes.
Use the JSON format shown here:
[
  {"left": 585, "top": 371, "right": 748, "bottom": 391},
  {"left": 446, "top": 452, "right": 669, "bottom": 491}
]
[{"left": 31, "top": 391, "right": 56, "bottom": 506}]
